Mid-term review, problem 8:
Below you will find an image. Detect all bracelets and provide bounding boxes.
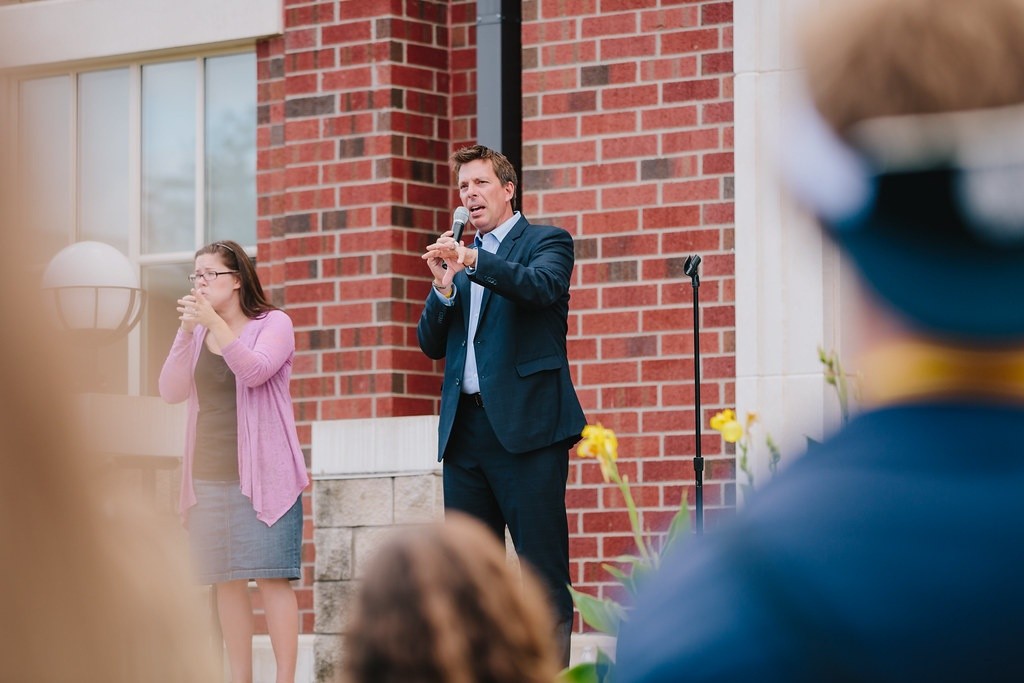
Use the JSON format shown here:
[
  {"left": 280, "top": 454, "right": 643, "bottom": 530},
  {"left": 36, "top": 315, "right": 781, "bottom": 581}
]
[{"left": 432, "top": 280, "right": 454, "bottom": 289}]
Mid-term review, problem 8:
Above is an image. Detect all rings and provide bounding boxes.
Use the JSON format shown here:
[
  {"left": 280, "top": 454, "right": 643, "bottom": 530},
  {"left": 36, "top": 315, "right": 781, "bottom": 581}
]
[
  {"left": 194, "top": 310, "right": 198, "bottom": 316},
  {"left": 449, "top": 244, "right": 456, "bottom": 250}
]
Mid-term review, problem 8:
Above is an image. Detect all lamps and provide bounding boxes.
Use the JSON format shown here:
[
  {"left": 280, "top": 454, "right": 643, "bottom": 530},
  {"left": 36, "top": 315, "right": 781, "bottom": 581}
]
[{"left": 37, "top": 240, "right": 147, "bottom": 394}]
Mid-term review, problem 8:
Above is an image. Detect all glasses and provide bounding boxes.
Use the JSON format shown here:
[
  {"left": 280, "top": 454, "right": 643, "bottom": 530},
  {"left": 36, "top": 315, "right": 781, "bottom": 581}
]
[{"left": 188, "top": 269, "right": 239, "bottom": 282}]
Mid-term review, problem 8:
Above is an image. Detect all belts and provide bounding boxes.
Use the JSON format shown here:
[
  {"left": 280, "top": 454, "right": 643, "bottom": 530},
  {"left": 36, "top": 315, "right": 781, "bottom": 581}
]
[{"left": 457, "top": 390, "right": 486, "bottom": 409}]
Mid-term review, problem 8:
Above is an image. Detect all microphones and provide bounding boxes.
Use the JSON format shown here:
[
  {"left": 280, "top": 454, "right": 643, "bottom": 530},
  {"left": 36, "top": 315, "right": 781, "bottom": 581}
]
[{"left": 442, "top": 206, "right": 468, "bottom": 271}]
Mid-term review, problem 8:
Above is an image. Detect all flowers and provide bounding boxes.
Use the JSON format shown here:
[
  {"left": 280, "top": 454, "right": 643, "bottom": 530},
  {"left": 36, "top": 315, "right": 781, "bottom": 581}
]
[{"left": 553, "top": 344, "right": 849, "bottom": 683}]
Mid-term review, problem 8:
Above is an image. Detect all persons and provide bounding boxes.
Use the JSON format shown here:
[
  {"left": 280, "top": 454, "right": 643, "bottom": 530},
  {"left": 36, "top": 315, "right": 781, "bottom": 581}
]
[
  {"left": 416, "top": 145, "right": 588, "bottom": 672},
  {"left": 612, "top": 1, "right": 1024, "bottom": 683},
  {"left": 158, "top": 241, "right": 309, "bottom": 683},
  {"left": 338, "top": 510, "right": 561, "bottom": 683}
]
